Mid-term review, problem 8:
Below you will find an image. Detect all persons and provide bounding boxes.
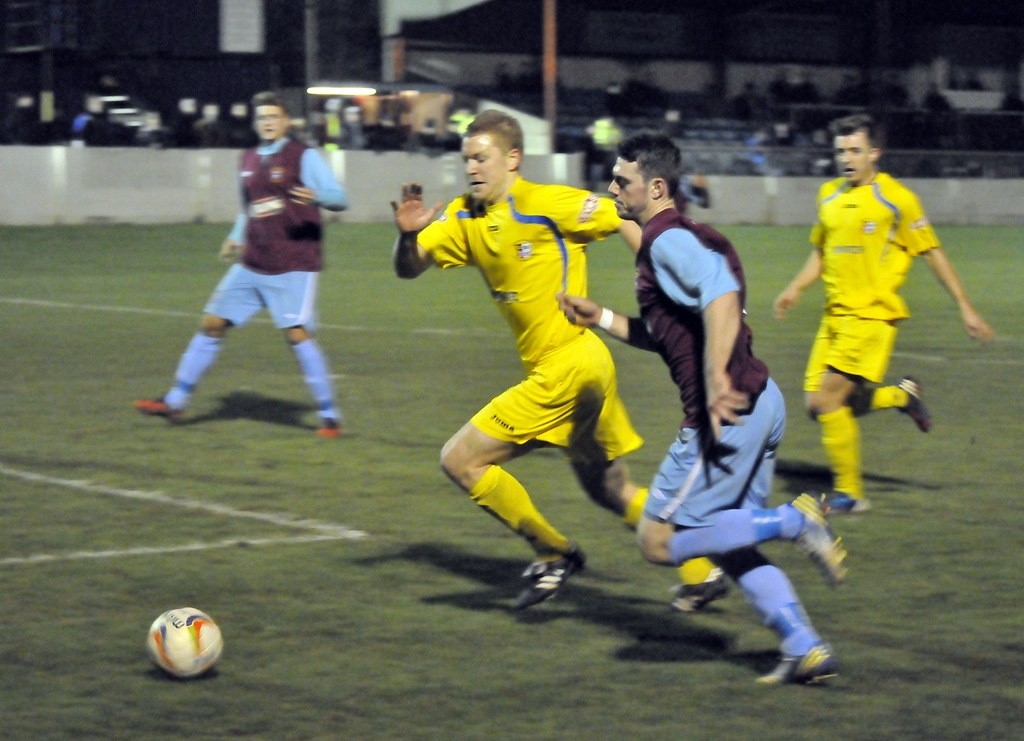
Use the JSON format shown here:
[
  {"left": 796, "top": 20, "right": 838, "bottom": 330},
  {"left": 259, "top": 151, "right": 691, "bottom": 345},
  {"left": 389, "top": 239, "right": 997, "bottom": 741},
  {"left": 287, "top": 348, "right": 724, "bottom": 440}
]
[
  {"left": 772, "top": 114, "right": 997, "bottom": 516},
  {"left": 391, "top": 112, "right": 732, "bottom": 614},
  {"left": 554, "top": 132, "right": 849, "bottom": 684},
  {"left": 1, "top": 60, "right": 1024, "bottom": 178},
  {"left": 133, "top": 89, "right": 351, "bottom": 438}
]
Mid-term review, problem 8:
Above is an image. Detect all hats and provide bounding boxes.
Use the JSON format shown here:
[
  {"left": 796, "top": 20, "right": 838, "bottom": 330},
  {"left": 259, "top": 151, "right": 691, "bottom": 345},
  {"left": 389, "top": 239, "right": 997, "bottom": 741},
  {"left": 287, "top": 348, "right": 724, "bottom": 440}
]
[{"left": 251, "top": 91, "right": 286, "bottom": 105}]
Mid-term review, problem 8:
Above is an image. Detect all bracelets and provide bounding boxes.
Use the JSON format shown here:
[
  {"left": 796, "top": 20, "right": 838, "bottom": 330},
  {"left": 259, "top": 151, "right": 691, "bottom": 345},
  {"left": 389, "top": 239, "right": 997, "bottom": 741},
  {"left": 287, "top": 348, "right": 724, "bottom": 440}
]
[{"left": 597, "top": 307, "right": 613, "bottom": 329}]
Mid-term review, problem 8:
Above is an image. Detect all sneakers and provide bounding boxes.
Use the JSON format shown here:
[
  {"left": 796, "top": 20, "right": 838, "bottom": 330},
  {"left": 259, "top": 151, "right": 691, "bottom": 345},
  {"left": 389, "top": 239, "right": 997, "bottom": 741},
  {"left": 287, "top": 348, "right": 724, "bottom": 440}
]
[
  {"left": 752, "top": 638, "right": 841, "bottom": 684},
  {"left": 513, "top": 541, "right": 587, "bottom": 610},
  {"left": 897, "top": 373, "right": 934, "bottom": 433},
  {"left": 669, "top": 566, "right": 733, "bottom": 613},
  {"left": 822, "top": 495, "right": 871, "bottom": 513},
  {"left": 134, "top": 397, "right": 185, "bottom": 419},
  {"left": 790, "top": 490, "right": 851, "bottom": 587},
  {"left": 313, "top": 426, "right": 347, "bottom": 436}
]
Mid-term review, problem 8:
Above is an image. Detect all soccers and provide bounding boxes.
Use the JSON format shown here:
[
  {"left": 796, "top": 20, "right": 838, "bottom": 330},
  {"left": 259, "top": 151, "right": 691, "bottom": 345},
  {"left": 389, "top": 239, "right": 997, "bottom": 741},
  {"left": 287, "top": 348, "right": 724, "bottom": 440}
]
[{"left": 146, "top": 607, "right": 223, "bottom": 678}]
